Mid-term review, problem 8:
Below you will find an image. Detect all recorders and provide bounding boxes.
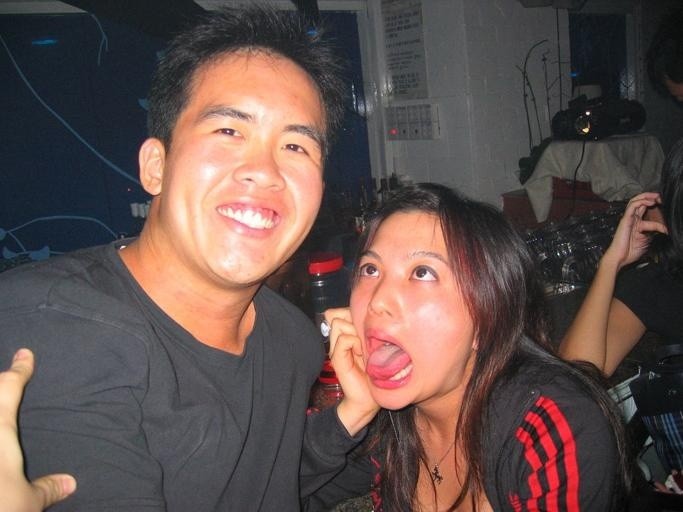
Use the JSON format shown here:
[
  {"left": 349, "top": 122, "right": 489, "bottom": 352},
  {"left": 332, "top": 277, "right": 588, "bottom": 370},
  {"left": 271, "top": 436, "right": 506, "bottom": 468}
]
[{"left": 551, "top": 95, "right": 646, "bottom": 139}]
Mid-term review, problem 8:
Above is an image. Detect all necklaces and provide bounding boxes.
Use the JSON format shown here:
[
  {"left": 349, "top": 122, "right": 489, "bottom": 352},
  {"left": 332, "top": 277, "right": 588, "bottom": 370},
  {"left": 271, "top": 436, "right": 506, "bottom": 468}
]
[{"left": 413, "top": 429, "right": 458, "bottom": 490}]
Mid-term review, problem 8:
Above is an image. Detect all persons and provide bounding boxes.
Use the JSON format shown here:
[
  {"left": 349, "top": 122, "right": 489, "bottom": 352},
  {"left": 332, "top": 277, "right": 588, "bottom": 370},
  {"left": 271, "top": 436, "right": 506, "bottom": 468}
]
[
  {"left": 0, "top": 344, "right": 77, "bottom": 511},
  {"left": 554, "top": 135, "right": 683, "bottom": 512},
  {"left": 293, "top": 178, "right": 640, "bottom": 512},
  {"left": 0, "top": 0, "right": 359, "bottom": 512},
  {"left": 641, "top": 1, "right": 682, "bottom": 106}
]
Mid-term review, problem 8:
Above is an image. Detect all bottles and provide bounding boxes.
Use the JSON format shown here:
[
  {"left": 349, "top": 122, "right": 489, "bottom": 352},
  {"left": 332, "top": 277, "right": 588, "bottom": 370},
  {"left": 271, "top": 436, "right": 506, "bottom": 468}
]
[{"left": 306, "top": 253, "right": 350, "bottom": 399}]
